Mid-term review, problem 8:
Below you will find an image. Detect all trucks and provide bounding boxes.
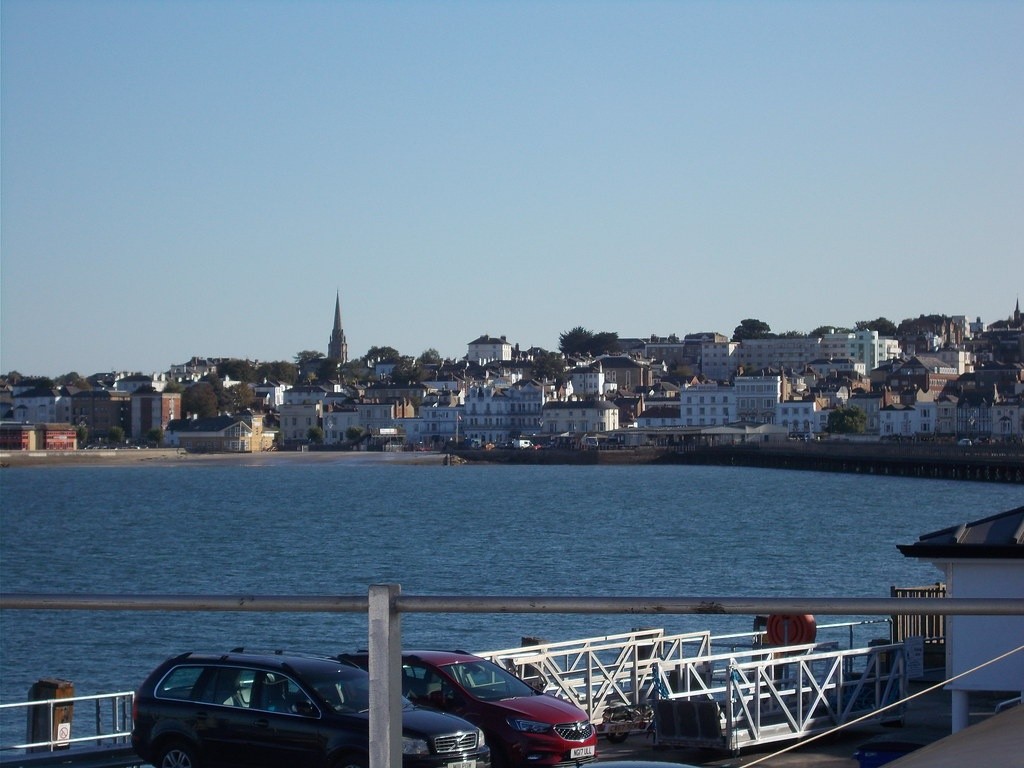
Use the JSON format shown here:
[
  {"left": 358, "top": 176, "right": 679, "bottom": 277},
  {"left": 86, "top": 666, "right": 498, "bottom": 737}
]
[{"left": 581, "top": 437, "right": 598, "bottom": 448}]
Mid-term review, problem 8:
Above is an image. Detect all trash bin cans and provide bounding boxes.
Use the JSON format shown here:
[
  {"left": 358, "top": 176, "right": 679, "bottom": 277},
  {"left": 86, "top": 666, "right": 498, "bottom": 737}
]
[
  {"left": 522, "top": 635, "right": 549, "bottom": 684},
  {"left": 25, "top": 678, "right": 75, "bottom": 752},
  {"left": 747, "top": 615, "right": 783, "bottom": 693},
  {"left": 853, "top": 741, "right": 925, "bottom": 768},
  {"left": 866, "top": 639, "right": 891, "bottom": 674},
  {"left": 632, "top": 627, "right": 664, "bottom": 683}
]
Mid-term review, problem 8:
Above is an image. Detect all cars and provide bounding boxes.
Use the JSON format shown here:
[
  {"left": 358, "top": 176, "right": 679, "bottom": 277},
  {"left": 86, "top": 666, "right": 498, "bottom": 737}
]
[{"left": 959, "top": 438, "right": 973, "bottom": 446}]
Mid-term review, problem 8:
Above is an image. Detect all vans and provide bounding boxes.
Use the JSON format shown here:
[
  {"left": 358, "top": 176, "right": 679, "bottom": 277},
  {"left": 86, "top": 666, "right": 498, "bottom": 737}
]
[{"left": 514, "top": 440, "right": 533, "bottom": 450}]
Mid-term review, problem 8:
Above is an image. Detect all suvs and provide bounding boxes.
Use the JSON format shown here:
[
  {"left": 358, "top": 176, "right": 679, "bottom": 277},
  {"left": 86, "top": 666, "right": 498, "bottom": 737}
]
[
  {"left": 333, "top": 649, "right": 600, "bottom": 768},
  {"left": 132, "top": 646, "right": 491, "bottom": 768}
]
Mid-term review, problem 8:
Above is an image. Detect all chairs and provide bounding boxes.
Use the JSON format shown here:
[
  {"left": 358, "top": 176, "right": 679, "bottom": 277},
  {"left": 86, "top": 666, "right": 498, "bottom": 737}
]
[
  {"left": 257, "top": 673, "right": 286, "bottom": 711},
  {"left": 424, "top": 670, "right": 442, "bottom": 700},
  {"left": 213, "top": 670, "right": 243, "bottom": 706}
]
